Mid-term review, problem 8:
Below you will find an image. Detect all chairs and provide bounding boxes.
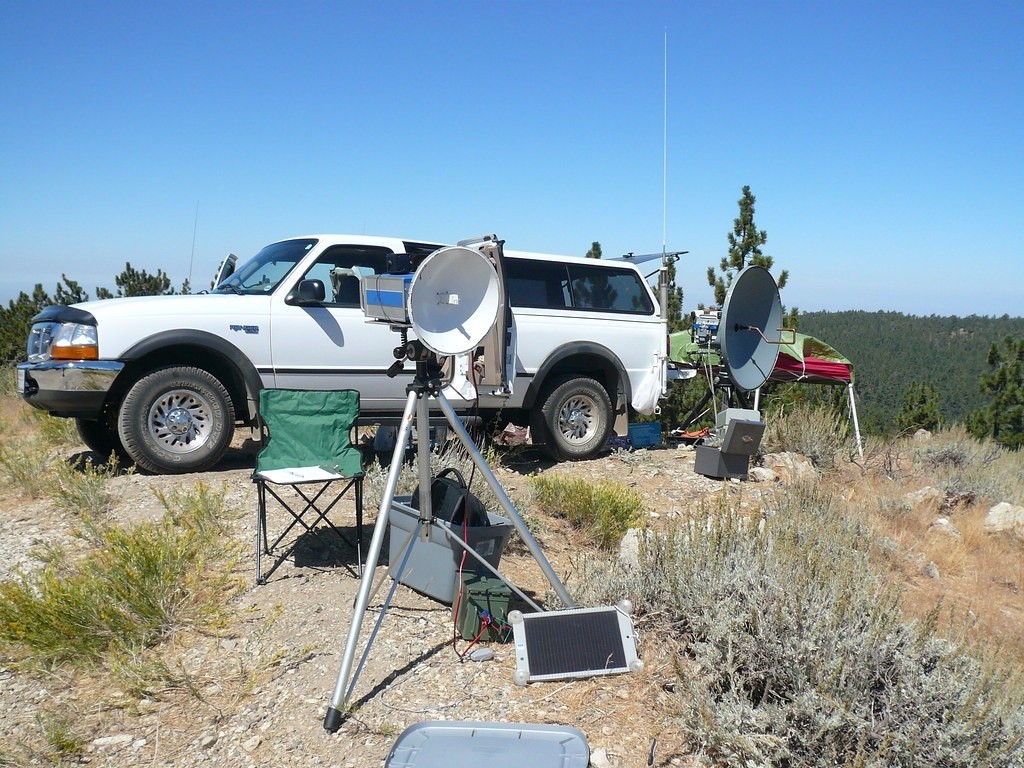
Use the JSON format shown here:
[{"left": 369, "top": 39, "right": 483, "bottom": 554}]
[
  {"left": 338, "top": 275, "right": 360, "bottom": 303},
  {"left": 250, "top": 388, "right": 367, "bottom": 586}
]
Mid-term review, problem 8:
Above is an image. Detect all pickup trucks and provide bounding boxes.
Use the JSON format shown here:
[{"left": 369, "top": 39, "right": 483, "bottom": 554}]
[{"left": 16, "top": 235, "right": 669, "bottom": 475}]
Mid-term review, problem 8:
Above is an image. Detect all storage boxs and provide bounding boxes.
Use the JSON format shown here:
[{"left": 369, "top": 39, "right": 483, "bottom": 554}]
[
  {"left": 694, "top": 420, "right": 765, "bottom": 481},
  {"left": 628, "top": 421, "right": 662, "bottom": 448},
  {"left": 386, "top": 495, "right": 515, "bottom": 604}
]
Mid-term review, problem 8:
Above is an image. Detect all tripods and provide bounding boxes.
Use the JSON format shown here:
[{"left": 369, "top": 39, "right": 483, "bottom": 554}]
[{"left": 323, "top": 340, "right": 576, "bottom": 731}]
